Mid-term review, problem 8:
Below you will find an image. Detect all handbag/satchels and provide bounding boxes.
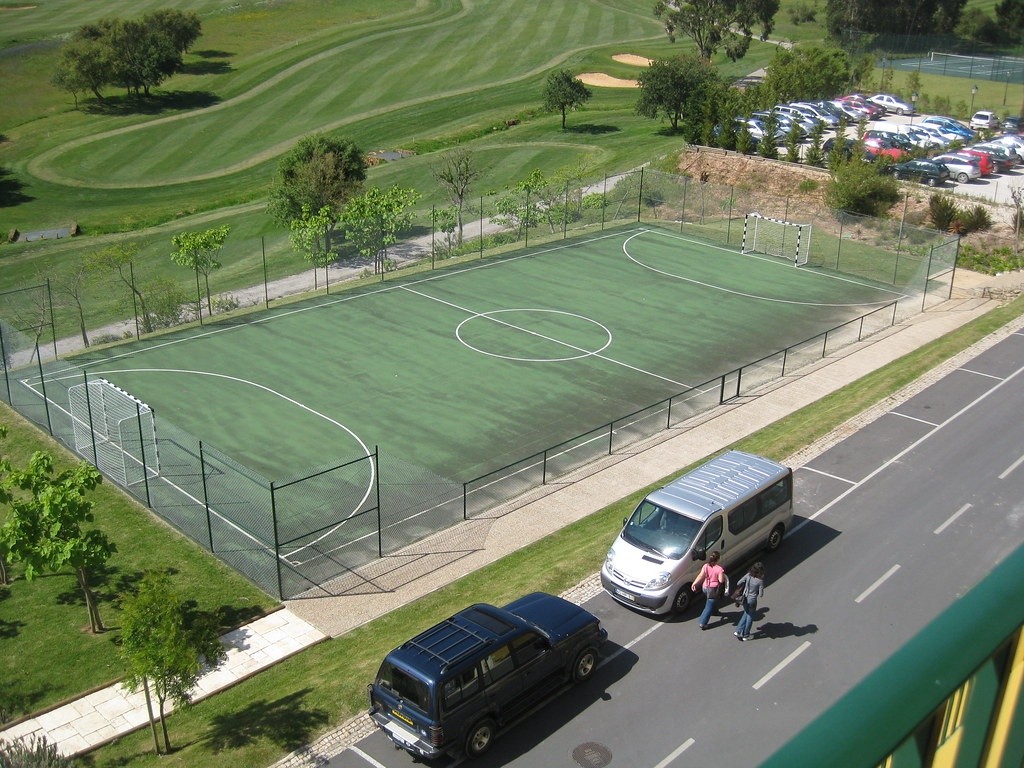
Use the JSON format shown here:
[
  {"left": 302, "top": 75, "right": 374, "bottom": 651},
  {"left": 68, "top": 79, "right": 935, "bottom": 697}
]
[
  {"left": 735, "top": 596, "right": 747, "bottom": 605},
  {"left": 707, "top": 587, "right": 722, "bottom": 599}
]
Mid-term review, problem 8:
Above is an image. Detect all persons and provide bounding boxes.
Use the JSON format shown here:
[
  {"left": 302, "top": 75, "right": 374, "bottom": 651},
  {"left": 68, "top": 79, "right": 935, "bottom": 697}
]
[
  {"left": 733, "top": 561, "right": 764, "bottom": 641},
  {"left": 691, "top": 551, "right": 725, "bottom": 629}
]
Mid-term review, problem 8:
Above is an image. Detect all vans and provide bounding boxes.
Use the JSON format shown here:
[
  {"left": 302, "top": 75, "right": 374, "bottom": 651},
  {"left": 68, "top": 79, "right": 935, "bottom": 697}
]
[{"left": 599, "top": 448, "right": 794, "bottom": 616}]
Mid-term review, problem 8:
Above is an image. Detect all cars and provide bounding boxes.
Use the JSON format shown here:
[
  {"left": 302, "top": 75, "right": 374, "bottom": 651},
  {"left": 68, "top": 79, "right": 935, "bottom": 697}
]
[
  {"left": 869, "top": 94, "right": 917, "bottom": 116},
  {"left": 969, "top": 111, "right": 1000, "bottom": 132},
  {"left": 1000, "top": 116, "right": 1024, "bottom": 135},
  {"left": 710, "top": 94, "right": 887, "bottom": 153},
  {"left": 819, "top": 115, "right": 1024, "bottom": 187}
]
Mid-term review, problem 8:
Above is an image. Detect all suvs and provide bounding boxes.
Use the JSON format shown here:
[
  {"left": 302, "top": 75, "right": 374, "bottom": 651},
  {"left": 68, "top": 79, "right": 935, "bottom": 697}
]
[{"left": 366, "top": 591, "right": 609, "bottom": 762}]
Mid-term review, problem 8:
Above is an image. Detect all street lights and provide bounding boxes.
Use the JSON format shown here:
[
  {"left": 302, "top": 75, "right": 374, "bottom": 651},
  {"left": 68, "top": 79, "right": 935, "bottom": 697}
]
[
  {"left": 881, "top": 57, "right": 886, "bottom": 94},
  {"left": 1003, "top": 72, "right": 1011, "bottom": 106},
  {"left": 909, "top": 92, "right": 919, "bottom": 133},
  {"left": 969, "top": 83, "right": 978, "bottom": 127}
]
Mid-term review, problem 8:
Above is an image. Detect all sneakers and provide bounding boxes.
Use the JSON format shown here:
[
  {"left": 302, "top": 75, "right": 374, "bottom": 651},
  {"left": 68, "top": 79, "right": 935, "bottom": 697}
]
[
  {"left": 733, "top": 631, "right": 742, "bottom": 640},
  {"left": 742, "top": 635, "right": 754, "bottom": 641},
  {"left": 699, "top": 624, "right": 707, "bottom": 630}
]
